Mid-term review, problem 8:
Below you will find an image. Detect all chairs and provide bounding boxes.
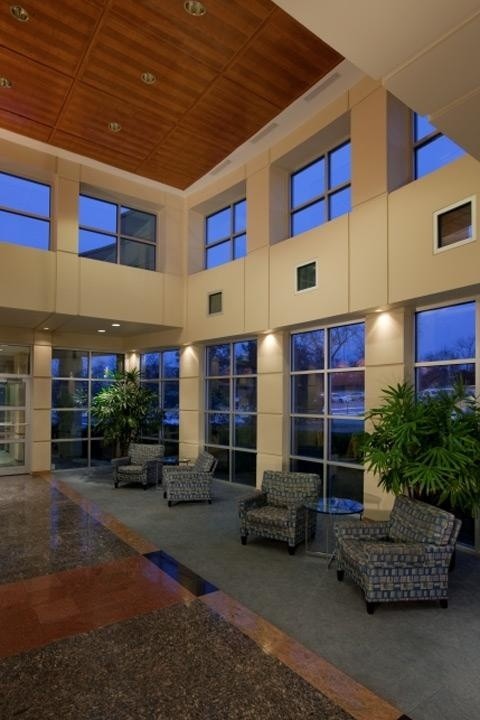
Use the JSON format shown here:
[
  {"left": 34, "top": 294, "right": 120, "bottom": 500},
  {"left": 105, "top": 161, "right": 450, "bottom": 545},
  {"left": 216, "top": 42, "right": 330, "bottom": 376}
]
[
  {"left": 161, "top": 451, "right": 219, "bottom": 508},
  {"left": 233, "top": 469, "right": 323, "bottom": 555},
  {"left": 110, "top": 443, "right": 166, "bottom": 491},
  {"left": 328, "top": 494, "right": 463, "bottom": 615}
]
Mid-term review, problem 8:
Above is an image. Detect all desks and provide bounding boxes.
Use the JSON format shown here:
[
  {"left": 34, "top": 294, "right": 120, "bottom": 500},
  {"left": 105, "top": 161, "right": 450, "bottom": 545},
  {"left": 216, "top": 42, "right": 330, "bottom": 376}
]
[
  {"left": 303, "top": 497, "right": 364, "bottom": 569},
  {"left": 155, "top": 456, "right": 192, "bottom": 491}
]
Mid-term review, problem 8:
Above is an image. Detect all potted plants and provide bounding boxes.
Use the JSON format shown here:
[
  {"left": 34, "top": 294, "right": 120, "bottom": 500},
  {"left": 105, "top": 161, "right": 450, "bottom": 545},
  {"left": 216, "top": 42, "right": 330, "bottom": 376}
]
[{"left": 349, "top": 373, "right": 480, "bottom": 571}]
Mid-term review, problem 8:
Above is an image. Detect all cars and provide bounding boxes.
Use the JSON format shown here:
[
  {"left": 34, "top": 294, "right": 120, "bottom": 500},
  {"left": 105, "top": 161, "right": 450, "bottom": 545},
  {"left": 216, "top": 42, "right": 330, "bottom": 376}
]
[
  {"left": 319, "top": 390, "right": 365, "bottom": 404},
  {"left": 418, "top": 386, "right": 478, "bottom": 423}
]
